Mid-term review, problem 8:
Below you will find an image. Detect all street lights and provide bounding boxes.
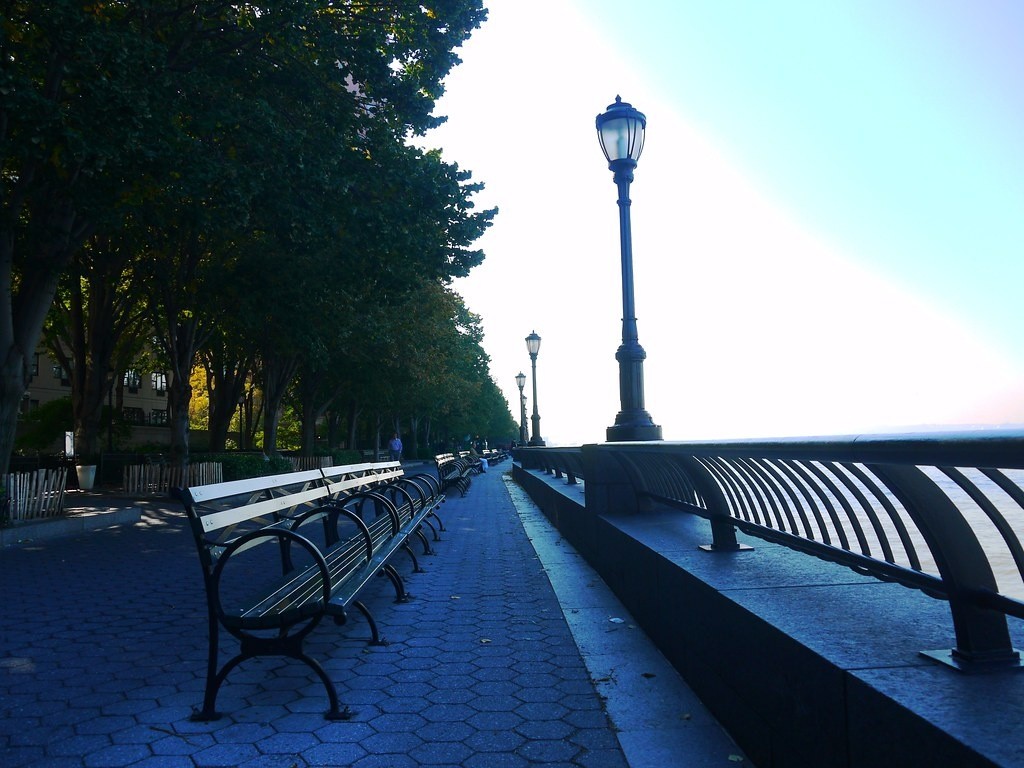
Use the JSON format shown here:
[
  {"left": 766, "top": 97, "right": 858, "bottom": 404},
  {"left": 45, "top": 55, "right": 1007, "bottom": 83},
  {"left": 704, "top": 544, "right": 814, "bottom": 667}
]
[
  {"left": 516, "top": 371, "right": 527, "bottom": 447},
  {"left": 596, "top": 95, "right": 662, "bottom": 442},
  {"left": 525, "top": 329, "right": 547, "bottom": 447}
]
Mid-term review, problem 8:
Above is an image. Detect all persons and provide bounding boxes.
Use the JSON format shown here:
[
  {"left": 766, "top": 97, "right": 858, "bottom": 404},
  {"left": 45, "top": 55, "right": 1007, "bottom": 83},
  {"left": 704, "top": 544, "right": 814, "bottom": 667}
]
[
  {"left": 388, "top": 432, "right": 402, "bottom": 461},
  {"left": 478, "top": 455, "right": 489, "bottom": 473}
]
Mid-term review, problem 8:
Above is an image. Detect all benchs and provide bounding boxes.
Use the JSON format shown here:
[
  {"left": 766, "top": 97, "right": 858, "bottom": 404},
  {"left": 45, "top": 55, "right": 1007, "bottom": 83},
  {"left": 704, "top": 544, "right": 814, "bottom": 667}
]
[{"left": 184, "top": 448, "right": 509, "bottom": 722}]
